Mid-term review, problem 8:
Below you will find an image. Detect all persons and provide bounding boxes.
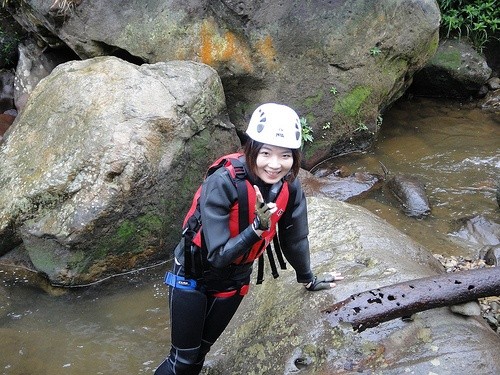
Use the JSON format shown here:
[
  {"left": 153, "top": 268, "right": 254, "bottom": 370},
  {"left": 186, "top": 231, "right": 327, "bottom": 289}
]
[{"left": 153, "top": 102, "right": 347, "bottom": 375}]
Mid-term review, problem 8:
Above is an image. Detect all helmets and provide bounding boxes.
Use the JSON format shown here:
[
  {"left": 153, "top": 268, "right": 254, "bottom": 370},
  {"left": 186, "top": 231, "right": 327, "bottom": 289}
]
[{"left": 248, "top": 103, "right": 304, "bottom": 148}]
